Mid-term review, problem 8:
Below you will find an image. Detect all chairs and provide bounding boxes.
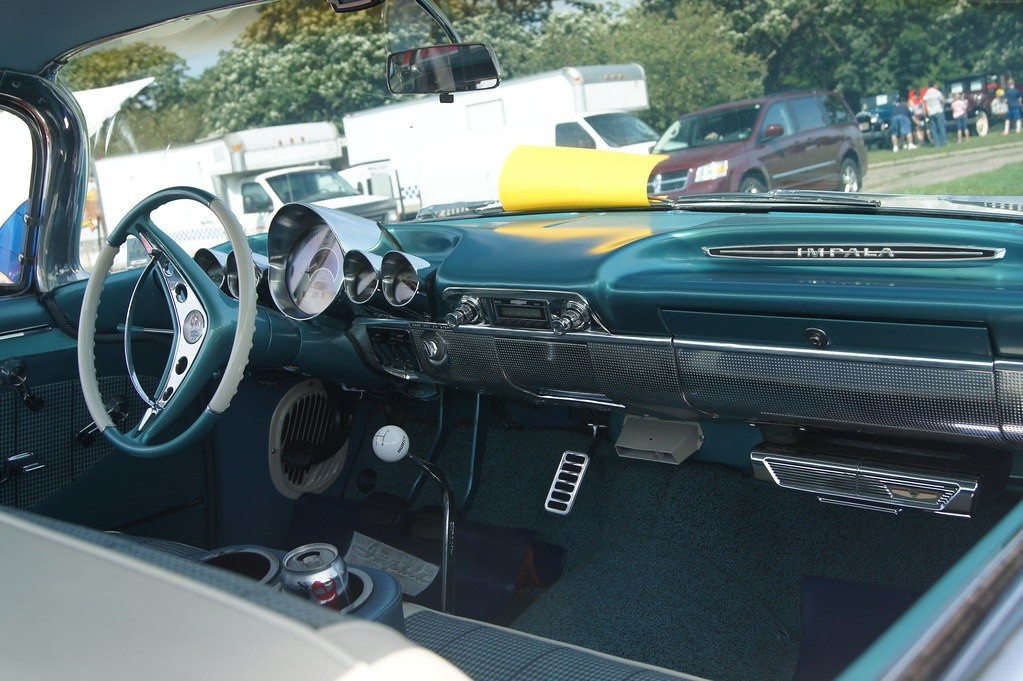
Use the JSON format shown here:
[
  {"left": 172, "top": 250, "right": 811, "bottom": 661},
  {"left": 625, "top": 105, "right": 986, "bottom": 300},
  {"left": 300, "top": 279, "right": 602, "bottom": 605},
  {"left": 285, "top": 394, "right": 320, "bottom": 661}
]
[{"left": 0, "top": 508, "right": 474, "bottom": 681}]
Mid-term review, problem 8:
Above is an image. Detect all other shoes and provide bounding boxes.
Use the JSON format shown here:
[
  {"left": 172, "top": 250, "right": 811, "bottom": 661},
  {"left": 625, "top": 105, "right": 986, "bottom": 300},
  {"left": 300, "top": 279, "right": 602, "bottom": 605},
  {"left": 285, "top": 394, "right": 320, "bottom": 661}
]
[{"left": 890, "top": 129, "right": 1022, "bottom": 153}]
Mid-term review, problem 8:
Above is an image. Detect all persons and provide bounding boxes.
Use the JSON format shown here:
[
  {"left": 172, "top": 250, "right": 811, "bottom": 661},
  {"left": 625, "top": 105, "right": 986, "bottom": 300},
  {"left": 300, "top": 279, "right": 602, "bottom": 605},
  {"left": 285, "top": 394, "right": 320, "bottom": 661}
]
[{"left": 883, "top": 67, "right": 1023, "bottom": 153}]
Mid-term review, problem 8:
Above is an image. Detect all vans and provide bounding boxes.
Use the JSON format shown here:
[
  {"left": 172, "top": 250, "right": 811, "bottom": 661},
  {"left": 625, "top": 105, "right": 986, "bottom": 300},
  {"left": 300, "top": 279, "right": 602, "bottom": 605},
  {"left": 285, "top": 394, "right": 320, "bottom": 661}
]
[{"left": 637, "top": 85, "right": 869, "bottom": 206}]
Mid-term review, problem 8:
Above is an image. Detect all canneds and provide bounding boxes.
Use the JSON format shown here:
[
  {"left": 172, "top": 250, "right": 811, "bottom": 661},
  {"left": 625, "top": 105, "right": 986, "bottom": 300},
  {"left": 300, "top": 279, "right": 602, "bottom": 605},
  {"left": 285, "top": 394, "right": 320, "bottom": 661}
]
[{"left": 279, "top": 543, "right": 352, "bottom": 611}]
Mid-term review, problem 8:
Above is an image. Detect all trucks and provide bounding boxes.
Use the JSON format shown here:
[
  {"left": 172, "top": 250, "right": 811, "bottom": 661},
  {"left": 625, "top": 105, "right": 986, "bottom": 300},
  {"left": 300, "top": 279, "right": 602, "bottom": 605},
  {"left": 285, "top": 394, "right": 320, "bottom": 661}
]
[
  {"left": 332, "top": 56, "right": 662, "bottom": 221},
  {"left": 88, "top": 119, "right": 401, "bottom": 252}
]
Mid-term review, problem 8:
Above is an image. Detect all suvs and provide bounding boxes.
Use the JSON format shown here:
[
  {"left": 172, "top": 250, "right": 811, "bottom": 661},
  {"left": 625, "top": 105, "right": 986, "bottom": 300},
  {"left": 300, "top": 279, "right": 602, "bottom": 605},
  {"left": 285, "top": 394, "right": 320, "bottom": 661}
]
[{"left": 934, "top": 70, "right": 1023, "bottom": 138}]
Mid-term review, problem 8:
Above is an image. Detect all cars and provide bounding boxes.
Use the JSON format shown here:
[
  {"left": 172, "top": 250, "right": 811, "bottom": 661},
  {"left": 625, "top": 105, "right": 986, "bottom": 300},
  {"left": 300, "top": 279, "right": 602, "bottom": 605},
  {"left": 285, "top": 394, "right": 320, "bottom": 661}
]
[{"left": 850, "top": 103, "right": 901, "bottom": 146}]
